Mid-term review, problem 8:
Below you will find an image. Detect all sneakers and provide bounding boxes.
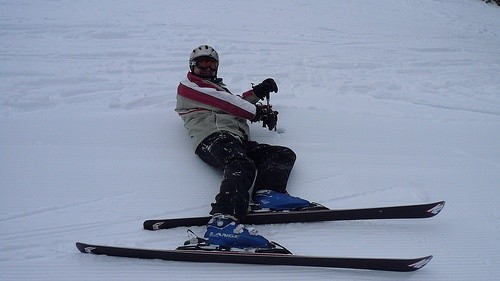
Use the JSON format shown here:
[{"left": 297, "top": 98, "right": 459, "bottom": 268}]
[
  {"left": 249, "top": 189, "right": 311, "bottom": 210},
  {"left": 203, "top": 212, "right": 273, "bottom": 249}
]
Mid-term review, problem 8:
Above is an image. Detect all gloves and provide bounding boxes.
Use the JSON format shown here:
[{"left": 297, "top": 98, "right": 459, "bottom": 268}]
[
  {"left": 251, "top": 105, "right": 279, "bottom": 131},
  {"left": 252, "top": 78, "right": 278, "bottom": 101}
]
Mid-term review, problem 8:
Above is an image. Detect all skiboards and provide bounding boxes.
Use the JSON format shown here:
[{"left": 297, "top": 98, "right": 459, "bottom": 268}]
[{"left": 75, "top": 199, "right": 445, "bottom": 272}]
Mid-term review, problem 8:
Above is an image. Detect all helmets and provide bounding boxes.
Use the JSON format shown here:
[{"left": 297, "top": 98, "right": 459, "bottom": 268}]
[{"left": 189, "top": 45, "right": 219, "bottom": 68}]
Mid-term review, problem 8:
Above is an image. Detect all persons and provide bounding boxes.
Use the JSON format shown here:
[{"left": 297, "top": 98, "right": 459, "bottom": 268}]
[{"left": 175, "top": 44, "right": 310, "bottom": 251}]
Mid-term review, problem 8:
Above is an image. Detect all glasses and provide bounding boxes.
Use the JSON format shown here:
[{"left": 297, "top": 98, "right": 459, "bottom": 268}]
[{"left": 195, "top": 58, "right": 218, "bottom": 70}]
